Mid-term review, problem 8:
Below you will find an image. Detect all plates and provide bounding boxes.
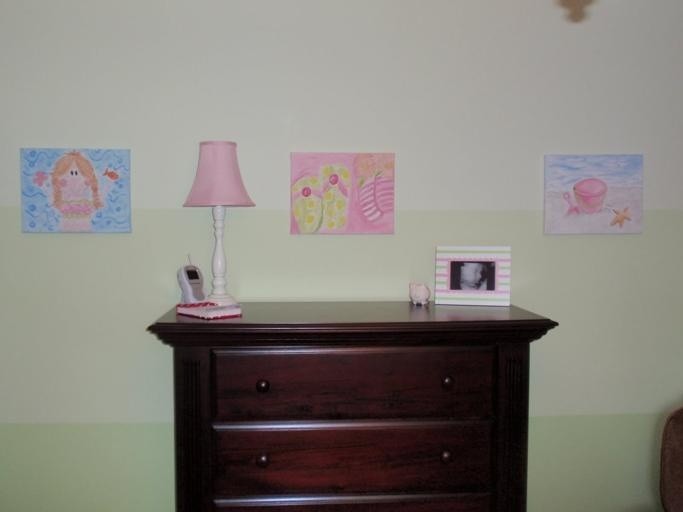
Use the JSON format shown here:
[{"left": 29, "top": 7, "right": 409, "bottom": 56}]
[{"left": 146, "top": 302, "right": 559, "bottom": 510}]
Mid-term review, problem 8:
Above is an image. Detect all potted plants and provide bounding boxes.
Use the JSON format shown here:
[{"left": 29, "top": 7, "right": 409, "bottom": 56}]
[{"left": 434, "top": 245, "right": 511, "bottom": 308}]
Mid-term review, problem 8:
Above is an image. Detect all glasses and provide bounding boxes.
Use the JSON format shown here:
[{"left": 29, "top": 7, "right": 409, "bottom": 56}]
[{"left": 184, "top": 140, "right": 255, "bottom": 308}]
